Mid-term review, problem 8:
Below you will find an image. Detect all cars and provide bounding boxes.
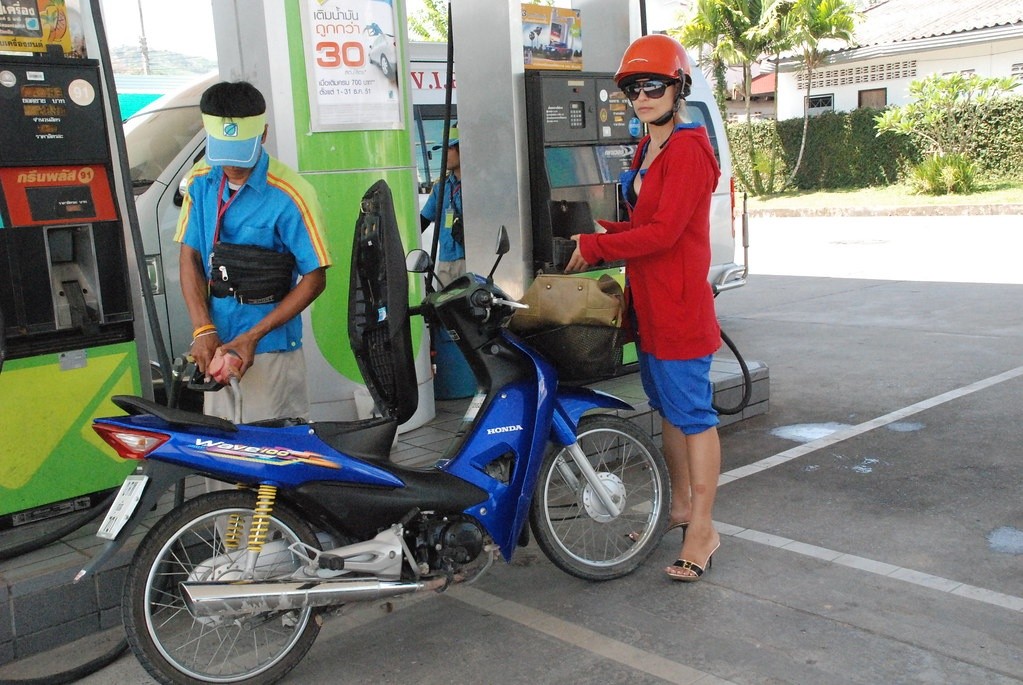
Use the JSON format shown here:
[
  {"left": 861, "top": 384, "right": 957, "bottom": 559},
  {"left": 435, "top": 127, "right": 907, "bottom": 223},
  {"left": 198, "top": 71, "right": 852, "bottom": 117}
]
[{"left": 366, "top": 33, "right": 397, "bottom": 82}]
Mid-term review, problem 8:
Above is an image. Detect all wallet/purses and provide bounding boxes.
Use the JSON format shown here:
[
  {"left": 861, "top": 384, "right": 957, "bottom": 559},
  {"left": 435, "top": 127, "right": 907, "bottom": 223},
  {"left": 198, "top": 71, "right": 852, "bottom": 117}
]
[{"left": 547, "top": 199, "right": 605, "bottom": 272}]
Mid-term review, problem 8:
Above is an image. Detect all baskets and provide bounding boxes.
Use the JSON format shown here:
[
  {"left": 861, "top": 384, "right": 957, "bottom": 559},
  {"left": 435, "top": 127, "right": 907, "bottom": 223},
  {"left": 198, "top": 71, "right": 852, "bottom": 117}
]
[{"left": 525, "top": 320, "right": 622, "bottom": 387}]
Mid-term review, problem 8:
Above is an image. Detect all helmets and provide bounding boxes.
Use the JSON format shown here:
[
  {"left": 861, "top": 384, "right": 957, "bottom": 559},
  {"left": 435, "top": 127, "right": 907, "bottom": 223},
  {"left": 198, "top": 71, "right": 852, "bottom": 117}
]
[{"left": 612, "top": 34, "right": 692, "bottom": 86}]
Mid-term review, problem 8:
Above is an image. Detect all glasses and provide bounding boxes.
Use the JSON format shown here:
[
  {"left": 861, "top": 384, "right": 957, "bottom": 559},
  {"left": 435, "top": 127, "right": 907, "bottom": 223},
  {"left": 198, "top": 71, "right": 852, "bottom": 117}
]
[{"left": 624, "top": 78, "right": 680, "bottom": 101}]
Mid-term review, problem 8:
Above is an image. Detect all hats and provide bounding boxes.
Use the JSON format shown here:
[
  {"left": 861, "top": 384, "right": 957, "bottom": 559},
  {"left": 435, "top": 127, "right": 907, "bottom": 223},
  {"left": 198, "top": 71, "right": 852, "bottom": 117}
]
[
  {"left": 201, "top": 111, "right": 266, "bottom": 168},
  {"left": 431, "top": 128, "right": 461, "bottom": 151}
]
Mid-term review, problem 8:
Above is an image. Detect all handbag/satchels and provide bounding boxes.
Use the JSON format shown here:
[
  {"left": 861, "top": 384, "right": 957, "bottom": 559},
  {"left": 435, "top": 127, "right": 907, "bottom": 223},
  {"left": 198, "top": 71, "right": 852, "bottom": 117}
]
[
  {"left": 505, "top": 272, "right": 626, "bottom": 332},
  {"left": 211, "top": 242, "right": 297, "bottom": 305},
  {"left": 451, "top": 212, "right": 464, "bottom": 247}
]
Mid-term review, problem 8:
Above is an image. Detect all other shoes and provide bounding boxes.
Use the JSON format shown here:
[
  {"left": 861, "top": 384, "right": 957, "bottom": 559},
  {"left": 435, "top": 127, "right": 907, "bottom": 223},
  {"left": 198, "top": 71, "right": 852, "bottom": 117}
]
[{"left": 282, "top": 608, "right": 323, "bottom": 628}]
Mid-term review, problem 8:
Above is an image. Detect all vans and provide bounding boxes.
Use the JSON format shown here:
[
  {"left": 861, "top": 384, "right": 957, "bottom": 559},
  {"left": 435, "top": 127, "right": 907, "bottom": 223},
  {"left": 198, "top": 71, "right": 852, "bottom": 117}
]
[{"left": 121, "top": 38, "right": 462, "bottom": 375}]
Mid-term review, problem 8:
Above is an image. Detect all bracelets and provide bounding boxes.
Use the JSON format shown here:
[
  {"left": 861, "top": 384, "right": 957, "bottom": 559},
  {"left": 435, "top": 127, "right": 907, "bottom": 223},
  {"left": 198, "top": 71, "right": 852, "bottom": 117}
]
[{"left": 190, "top": 325, "right": 218, "bottom": 348}]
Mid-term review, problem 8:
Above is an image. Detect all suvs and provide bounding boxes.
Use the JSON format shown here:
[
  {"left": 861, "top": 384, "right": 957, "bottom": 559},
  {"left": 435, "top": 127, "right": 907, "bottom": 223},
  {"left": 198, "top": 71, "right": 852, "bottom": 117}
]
[
  {"left": 544, "top": 41, "right": 573, "bottom": 60},
  {"left": 679, "top": 60, "right": 749, "bottom": 293}
]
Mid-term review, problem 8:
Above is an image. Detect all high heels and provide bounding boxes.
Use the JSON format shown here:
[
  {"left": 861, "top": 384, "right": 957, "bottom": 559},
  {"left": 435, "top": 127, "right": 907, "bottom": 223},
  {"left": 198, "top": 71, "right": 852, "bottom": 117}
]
[
  {"left": 667, "top": 540, "right": 720, "bottom": 581},
  {"left": 628, "top": 521, "right": 690, "bottom": 543}
]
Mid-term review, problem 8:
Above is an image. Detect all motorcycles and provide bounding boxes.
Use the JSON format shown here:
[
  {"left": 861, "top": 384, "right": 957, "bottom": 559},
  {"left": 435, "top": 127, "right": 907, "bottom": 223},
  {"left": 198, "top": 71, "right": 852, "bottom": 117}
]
[{"left": 66, "top": 223, "right": 673, "bottom": 685}]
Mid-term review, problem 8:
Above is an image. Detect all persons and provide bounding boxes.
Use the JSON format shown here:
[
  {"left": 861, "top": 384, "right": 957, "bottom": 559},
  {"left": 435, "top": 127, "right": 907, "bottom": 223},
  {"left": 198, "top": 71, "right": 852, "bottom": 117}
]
[
  {"left": 420, "top": 121, "right": 462, "bottom": 374},
  {"left": 168, "top": 81, "right": 332, "bottom": 627},
  {"left": 563, "top": 33, "right": 721, "bottom": 580}
]
